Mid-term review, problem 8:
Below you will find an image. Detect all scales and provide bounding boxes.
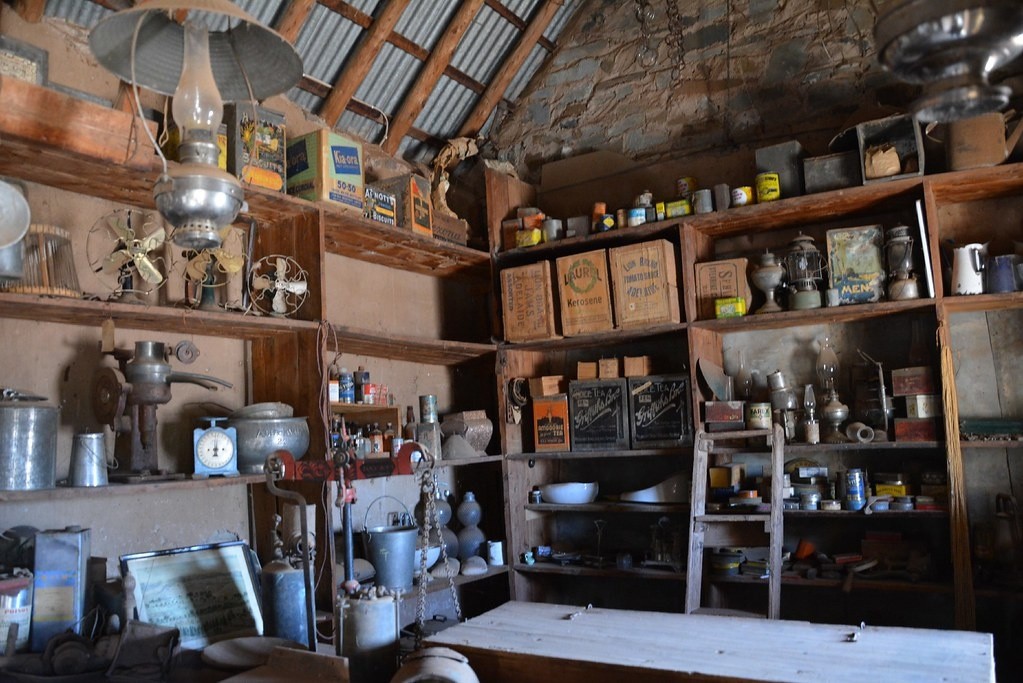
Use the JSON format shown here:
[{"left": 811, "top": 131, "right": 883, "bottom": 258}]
[{"left": 192, "top": 417, "right": 240, "bottom": 480}]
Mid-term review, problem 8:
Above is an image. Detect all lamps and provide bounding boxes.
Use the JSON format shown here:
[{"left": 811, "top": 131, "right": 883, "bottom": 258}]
[
  {"left": 88, "top": 0, "right": 303, "bottom": 249},
  {"left": 867, "top": 0, "right": 1023, "bottom": 122}
]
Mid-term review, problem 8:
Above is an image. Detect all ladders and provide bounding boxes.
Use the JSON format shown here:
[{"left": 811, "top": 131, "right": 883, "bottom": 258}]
[{"left": 684, "top": 423, "right": 785, "bottom": 620}]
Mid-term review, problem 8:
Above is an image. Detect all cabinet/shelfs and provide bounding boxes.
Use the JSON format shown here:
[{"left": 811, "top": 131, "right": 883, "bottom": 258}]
[{"left": 0, "top": 69, "right": 1023, "bottom": 683}]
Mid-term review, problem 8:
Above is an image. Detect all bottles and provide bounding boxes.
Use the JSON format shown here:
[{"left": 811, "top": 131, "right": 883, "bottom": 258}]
[
  {"left": 339, "top": 373, "right": 354, "bottom": 404},
  {"left": 404, "top": 405, "right": 417, "bottom": 441},
  {"left": 331, "top": 420, "right": 395, "bottom": 453},
  {"left": 531, "top": 491, "right": 542, "bottom": 503},
  {"left": 782, "top": 468, "right": 947, "bottom": 510}
]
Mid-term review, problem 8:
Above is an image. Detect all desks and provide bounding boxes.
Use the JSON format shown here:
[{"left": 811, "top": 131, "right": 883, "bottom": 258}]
[{"left": 419, "top": 600, "right": 996, "bottom": 683}]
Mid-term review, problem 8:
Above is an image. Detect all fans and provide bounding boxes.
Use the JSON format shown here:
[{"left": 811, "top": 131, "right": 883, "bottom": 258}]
[
  {"left": 167, "top": 224, "right": 245, "bottom": 313},
  {"left": 246, "top": 255, "right": 309, "bottom": 319},
  {"left": 87, "top": 208, "right": 174, "bottom": 306}
]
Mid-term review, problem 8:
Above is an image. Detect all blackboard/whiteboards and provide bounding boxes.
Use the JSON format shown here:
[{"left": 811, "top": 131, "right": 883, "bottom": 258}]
[
  {"left": 568, "top": 378, "right": 630, "bottom": 451},
  {"left": 628, "top": 373, "right": 694, "bottom": 448}
]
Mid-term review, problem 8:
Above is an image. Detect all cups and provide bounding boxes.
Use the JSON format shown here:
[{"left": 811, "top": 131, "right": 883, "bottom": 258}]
[
  {"left": 617, "top": 553, "right": 633, "bottom": 570},
  {"left": 951, "top": 241, "right": 991, "bottom": 296},
  {"left": 486, "top": 538, "right": 508, "bottom": 566},
  {"left": 985, "top": 254, "right": 1021, "bottom": 294}
]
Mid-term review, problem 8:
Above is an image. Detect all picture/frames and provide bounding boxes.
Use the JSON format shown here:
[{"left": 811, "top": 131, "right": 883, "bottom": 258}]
[{"left": 117, "top": 540, "right": 266, "bottom": 655}]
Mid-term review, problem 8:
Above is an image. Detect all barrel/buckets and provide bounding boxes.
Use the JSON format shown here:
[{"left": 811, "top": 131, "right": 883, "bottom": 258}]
[
  {"left": 362, "top": 495, "right": 419, "bottom": 595},
  {"left": 419, "top": 394, "right": 438, "bottom": 423},
  {"left": 390, "top": 438, "right": 404, "bottom": 458},
  {"left": 68, "top": 430, "right": 119, "bottom": 487},
  {"left": 596, "top": 171, "right": 782, "bottom": 232},
  {"left": 0, "top": 386, "right": 61, "bottom": 490}
]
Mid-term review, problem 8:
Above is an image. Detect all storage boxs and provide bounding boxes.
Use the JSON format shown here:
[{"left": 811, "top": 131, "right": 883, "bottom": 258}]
[
  {"left": 364, "top": 184, "right": 397, "bottom": 226},
  {"left": 578, "top": 361, "right": 597, "bottom": 378},
  {"left": 286, "top": 127, "right": 365, "bottom": 212},
  {"left": 529, "top": 375, "right": 568, "bottom": 397},
  {"left": 568, "top": 378, "right": 630, "bottom": 452},
  {"left": 555, "top": 249, "right": 616, "bottom": 337},
  {"left": 695, "top": 258, "right": 754, "bottom": 320},
  {"left": 609, "top": 239, "right": 681, "bottom": 329},
  {"left": 625, "top": 356, "right": 661, "bottom": 376},
  {"left": 368, "top": 172, "right": 432, "bottom": 238},
  {"left": 599, "top": 359, "right": 623, "bottom": 379},
  {"left": 628, "top": 374, "right": 695, "bottom": 448},
  {"left": 432, "top": 209, "right": 467, "bottom": 246},
  {"left": 222, "top": 101, "right": 286, "bottom": 194},
  {"left": 154, "top": 116, "right": 228, "bottom": 174},
  {"left": 532, "top": 393, "right": 570, "bottom": 453},
  {"left": 31, "top": 525, "right": 91, "bottom": 654},
  {"left": 500, "top": 260, "right": 563, "bottom": 342}
]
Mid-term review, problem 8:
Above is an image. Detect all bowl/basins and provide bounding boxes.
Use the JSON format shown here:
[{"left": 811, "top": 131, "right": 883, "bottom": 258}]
[
  {"left": 414, "top": 544, "right": 441, "bottom": 575},
  {"left": 226, "top": 416, "right": 310, "bottom": 473},
  {"left": 1, "top": 179, "right": 30, "bottom": 252}
]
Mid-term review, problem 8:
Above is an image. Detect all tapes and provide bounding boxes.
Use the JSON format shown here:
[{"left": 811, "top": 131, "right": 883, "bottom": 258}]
[{"left": 739, "top": 489, "right": 758, "bottom": 498}]
